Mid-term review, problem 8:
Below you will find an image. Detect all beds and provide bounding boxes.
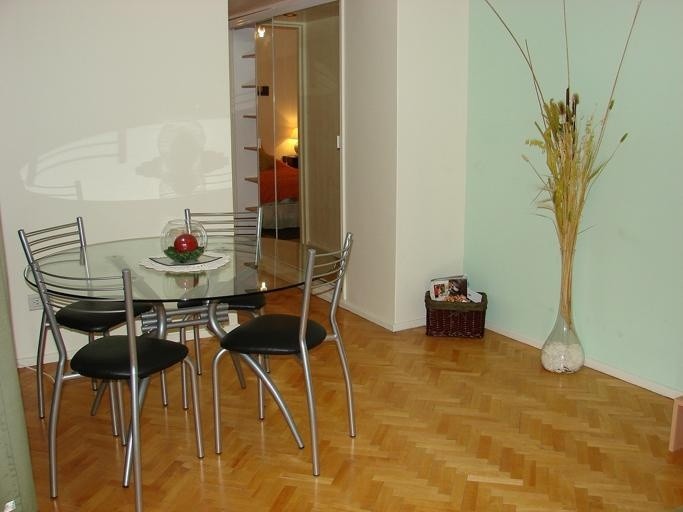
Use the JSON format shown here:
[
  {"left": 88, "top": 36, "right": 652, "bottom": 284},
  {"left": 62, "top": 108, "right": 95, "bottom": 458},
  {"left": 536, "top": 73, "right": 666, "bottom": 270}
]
[{"left": 259, "top": 149, "right": 299, "bottom": 239}]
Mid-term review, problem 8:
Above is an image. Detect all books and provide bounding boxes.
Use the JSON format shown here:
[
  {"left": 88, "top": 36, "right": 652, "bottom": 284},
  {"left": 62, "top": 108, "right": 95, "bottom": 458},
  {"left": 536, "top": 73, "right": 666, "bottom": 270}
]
[{"left": 430, "top": 275, "right": 467, "bottom": 302}]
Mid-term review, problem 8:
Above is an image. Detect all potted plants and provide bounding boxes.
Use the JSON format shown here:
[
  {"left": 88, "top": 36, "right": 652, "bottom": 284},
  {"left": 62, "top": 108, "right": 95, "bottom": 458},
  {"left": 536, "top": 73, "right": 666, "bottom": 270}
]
[{"left": 483, "top": 0, "right": 644, "bottom": 374}]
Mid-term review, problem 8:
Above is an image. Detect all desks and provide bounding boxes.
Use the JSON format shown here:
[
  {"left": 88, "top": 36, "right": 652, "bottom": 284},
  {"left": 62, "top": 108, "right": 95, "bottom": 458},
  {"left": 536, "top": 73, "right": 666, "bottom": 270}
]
[{"left": 22, "top": 235, "right": 342, "bottom": 488}]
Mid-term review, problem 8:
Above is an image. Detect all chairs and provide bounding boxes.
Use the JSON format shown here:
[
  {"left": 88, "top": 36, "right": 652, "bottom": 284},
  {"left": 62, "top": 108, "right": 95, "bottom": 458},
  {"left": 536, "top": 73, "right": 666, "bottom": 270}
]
[
  {"left": 210, "top": 233, "right": 357, "bottom": 477},
  {"left": 167, "top": 206, "right": 264, "bottom": 410},
  {"left": 31, "top": 260, "right": 203, "bottom": 512},
  {"left": 19, "top": 217, "right": 167, "bottom": 417}
]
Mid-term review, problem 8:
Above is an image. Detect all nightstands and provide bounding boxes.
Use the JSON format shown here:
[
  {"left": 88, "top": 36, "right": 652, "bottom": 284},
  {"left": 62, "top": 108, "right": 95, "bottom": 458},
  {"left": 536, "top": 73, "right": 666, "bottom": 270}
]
[{"left": 282, "top": 156, "right": 298, "bottom": 169}]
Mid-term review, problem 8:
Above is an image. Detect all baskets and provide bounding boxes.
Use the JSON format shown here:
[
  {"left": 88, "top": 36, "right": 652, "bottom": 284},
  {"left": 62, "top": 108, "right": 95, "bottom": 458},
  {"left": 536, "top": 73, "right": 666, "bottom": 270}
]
[{"left": 424, "top": 291, "right": 488, "bottom": 339}]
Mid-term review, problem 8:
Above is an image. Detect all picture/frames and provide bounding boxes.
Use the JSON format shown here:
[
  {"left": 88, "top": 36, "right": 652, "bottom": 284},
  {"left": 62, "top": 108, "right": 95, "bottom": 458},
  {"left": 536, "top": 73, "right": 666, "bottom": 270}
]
[{"left": 431, "top": 280, "right": 449, "bottom": 302}]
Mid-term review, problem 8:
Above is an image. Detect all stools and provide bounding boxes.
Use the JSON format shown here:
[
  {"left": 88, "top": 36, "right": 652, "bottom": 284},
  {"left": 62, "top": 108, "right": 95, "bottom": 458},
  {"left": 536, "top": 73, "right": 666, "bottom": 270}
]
[{"left": 668, "top": 394, "right": 683, "bottom": 451}]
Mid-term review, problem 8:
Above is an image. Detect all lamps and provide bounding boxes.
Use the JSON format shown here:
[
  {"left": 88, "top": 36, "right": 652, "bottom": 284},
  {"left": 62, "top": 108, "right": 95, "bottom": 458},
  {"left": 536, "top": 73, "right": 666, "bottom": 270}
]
[
  {"left": 258, "top": 24, "right": 266, "bottom": 38},
  {"left": 289, "top": 128, "right": 298, "bottom": 158}
]
